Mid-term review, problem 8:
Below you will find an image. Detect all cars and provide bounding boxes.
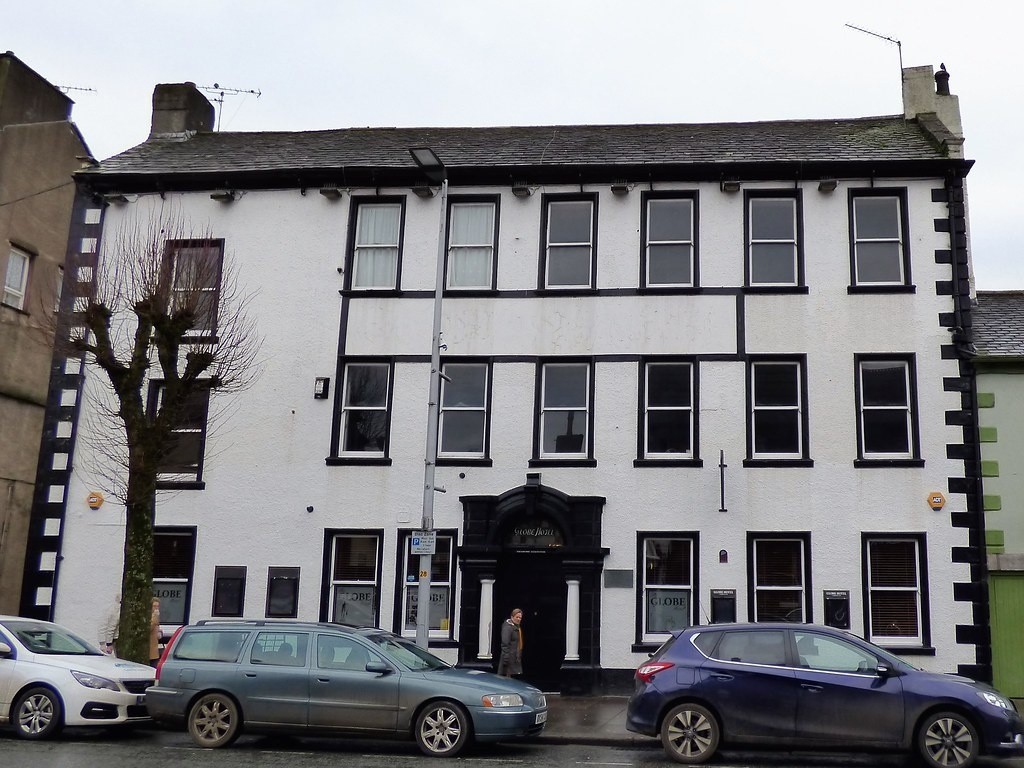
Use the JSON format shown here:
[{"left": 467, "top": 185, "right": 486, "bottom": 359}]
[
  {"left": 143, "top": 617, "right": 549, "bottom": 757},
  {"left": 0, "top": 614, "right": 158, "bottom": 740},
  {"left": 625, "top": 620, "right": 1024, "bottom": 768}
]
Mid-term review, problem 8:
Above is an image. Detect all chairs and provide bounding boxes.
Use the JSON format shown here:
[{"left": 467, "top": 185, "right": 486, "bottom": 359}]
[
  {"left": 251, "top": 643, "right": 266, "bottom": 664},
  {"left": 320, "top": 645, "right": 341, "bottom": 669},
  {"left": 347, "top": 643, "right": 372, "bottom": 670},
  {"left": 275, "top": 643, "right": 296, "bottom": 665}
]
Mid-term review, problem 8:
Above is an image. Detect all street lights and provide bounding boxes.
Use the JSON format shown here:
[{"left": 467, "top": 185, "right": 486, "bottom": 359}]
[{"left": 405, "top": 147, "right": 451, "bottom": 662}]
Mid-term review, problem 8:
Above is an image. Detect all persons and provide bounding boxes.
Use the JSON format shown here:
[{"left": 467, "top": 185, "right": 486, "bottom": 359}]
[
  {"left": 498, "top": 609, "right": 522, "bottom": 677},
  {"left": 149, "top": 598, "right": 163, "bottom": 665},
  {"left": 96, "top": 602, "right": 120, "bottom": 658}
]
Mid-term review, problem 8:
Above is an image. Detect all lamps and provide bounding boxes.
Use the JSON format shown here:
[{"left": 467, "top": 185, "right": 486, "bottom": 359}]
[
  {"left": 209, "top": 193, "right": 235, "bottom": 205},
  {"left": 319, "top": 188, "right": 344, "bottom": 201},
  {"left": 100, "top": 194, "right": 128, "bottom": 207},
  {"left": 611, "top": 184, "right": 630, "bottom": 196},
  {"left": 511, "top": 186, "right": 531, "bottom": 199},
  {"left": 411, "top": 185, "right": 434, "bottom": 200},
  {"left": 721, "top": 179, "right": 741, "bottom": 194},
  {"left": 818, "top": 179, "right": 837, "bottom": 194}
]
